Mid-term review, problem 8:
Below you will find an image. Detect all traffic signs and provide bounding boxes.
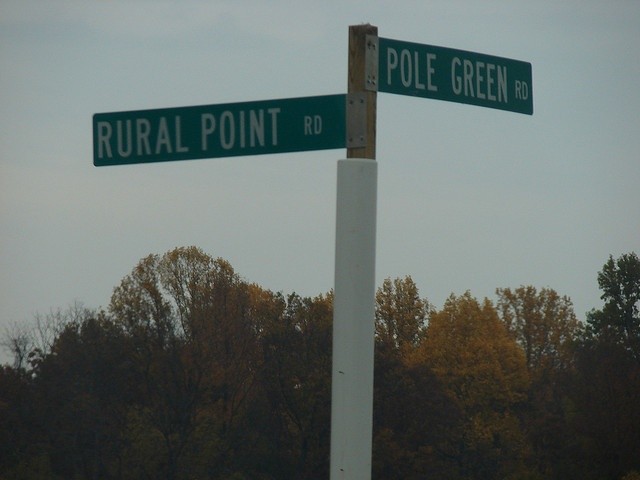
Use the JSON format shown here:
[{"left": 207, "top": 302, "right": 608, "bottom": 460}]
[
  {"left": 93, "top": 94, "right": 346, "bottom": 166},
  {"left": 378, "top": 37, "right": 534, "bottom": 115}
]
